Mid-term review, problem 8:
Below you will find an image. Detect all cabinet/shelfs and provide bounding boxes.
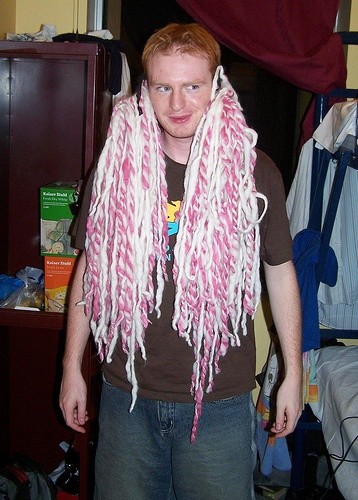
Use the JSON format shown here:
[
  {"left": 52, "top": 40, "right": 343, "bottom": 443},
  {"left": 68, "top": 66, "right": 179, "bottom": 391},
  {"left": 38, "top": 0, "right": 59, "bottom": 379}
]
[{"left": 0, "top": 40, "right": 106, "bottom": 500}]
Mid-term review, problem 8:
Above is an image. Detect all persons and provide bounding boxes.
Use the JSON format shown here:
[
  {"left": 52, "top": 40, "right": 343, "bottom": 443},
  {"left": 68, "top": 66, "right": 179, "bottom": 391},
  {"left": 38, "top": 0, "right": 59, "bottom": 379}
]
[{"left": 59, "top": 24, "right": 305, "bottom": 500}]
